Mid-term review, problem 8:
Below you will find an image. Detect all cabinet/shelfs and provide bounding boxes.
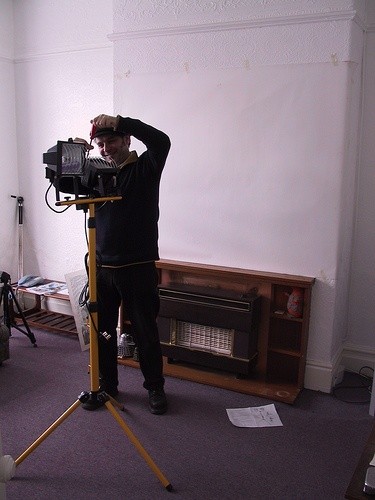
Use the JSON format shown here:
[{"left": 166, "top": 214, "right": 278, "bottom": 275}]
[{"left": 118, "top": 259, "right": 316, "bottom": 405}]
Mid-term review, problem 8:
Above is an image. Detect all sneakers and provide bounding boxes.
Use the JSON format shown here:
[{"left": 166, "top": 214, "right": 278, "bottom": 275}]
[
  {"left": 148, "top": 388, "right": 168, "bottom": 414},
  {"left": 80, "top": 388, "right": 119, "bottom": 409}
]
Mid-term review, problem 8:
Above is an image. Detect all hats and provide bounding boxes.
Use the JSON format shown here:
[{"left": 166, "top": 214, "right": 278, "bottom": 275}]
[{"left": 90, "top": 127, "right": 117, "bottom": 138}]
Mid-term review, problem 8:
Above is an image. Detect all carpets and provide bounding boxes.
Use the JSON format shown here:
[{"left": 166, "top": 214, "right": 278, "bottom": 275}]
[{"left": 0, "top": 321, "right": 375, "bottom": 500}]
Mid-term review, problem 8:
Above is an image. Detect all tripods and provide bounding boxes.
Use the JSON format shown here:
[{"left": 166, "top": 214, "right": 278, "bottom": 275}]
[
  {"left": 15, "top": 197, "right": 173, "bottom": 492},
  {"left": 0, "top": 271, "right": 37, "bottom": 348}
]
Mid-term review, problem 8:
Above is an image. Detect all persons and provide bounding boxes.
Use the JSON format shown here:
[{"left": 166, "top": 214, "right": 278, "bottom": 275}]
[{"left": 74, "top": 114, "right": 172, "bottom": 415}]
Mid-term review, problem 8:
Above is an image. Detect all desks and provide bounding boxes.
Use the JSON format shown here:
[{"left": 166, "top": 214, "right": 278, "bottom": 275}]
[{"left": 10, "top": 282, "right": 78, "bottom": 336}]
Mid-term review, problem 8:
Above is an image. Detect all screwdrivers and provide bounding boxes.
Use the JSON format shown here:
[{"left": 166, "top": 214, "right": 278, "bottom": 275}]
[{"left": 87, "top": 123, "right": 97, "bottom": 158}]
